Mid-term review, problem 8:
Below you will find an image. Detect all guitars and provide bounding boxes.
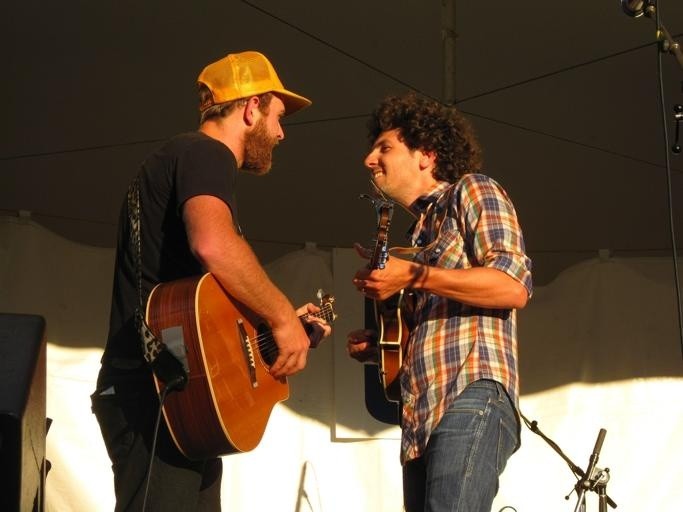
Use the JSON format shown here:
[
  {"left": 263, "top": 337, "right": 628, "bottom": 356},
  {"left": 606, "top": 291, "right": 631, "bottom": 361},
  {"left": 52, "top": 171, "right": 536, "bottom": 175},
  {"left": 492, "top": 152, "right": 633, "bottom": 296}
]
[
  {"left": 364, "top": 194, "right": 417, "bottom": 425},
  {"left": 145, "top": 272, "right": 338, "bottom": 461}
]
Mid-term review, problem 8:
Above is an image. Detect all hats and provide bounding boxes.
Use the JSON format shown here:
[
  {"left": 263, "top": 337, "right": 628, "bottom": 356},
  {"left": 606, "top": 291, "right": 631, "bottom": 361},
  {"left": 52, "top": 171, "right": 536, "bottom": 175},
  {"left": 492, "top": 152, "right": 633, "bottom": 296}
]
[{"left": 198, "top": 51, "right": 313, "bottom": 117}]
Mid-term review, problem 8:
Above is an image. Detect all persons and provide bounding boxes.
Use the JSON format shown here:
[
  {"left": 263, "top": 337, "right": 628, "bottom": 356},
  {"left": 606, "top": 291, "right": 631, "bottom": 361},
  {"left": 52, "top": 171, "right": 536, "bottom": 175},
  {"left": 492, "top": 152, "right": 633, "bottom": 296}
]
[
  {"left": 89, "top": 52, "right": 332, "bottom": 511},
  {"left": 345, "top": 91, "right": 533, "bottom": 511}
]
